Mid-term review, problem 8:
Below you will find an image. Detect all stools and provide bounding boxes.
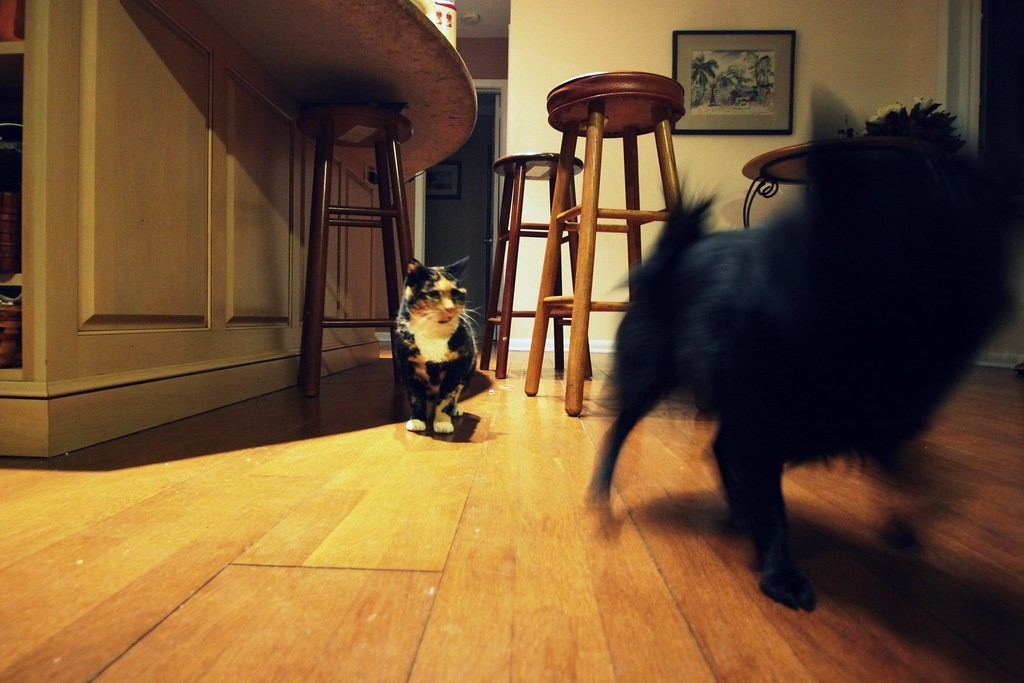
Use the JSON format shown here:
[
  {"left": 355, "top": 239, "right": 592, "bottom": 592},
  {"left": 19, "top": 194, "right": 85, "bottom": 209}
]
[
  {"left": 478, "top": 153, "right": 599, "bottom": 383},
  {"left": 524, "top": 72, "right": 706, "bottom": 414},
  {"left": 292, "top": 100, "right": 428, "bottom": 401}
]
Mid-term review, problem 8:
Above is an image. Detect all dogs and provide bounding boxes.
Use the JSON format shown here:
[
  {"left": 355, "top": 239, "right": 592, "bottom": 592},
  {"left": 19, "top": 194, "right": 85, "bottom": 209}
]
[{"left": 587, "top": 101, "right": 1011, "bottom": 614}]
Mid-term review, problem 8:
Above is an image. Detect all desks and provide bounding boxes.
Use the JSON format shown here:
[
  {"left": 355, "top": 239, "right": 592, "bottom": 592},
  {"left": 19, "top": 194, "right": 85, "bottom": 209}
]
[{"left": 740, "top": 136, "right": 941, "bottom": 235}]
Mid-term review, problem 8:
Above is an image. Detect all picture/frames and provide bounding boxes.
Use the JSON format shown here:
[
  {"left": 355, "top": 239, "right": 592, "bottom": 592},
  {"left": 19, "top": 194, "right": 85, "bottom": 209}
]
[{"left": 669, "top": 28, "right": 797, "bottom": 135}]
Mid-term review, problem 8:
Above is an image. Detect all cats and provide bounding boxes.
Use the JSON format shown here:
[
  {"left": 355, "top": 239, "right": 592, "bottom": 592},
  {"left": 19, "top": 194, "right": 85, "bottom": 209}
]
[{"left": 394, "top": 251, "right": 478, "bottom": 434}]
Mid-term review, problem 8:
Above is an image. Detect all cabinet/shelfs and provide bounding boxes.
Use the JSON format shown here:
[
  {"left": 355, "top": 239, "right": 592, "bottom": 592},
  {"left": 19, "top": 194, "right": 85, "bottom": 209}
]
[{"left": 0, "top": 0, "right": 479, "bottom": 456}]
